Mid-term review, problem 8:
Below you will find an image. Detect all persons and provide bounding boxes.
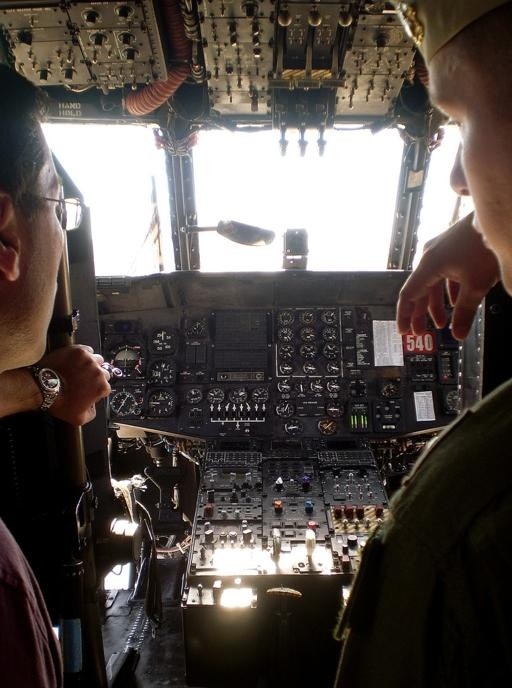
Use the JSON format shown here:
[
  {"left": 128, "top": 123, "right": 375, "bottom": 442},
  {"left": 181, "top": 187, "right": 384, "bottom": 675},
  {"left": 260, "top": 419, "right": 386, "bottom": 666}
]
[
  {"left": 0, "top": 62, "right": 112, "bottom": 688},
  {"left": 325, "top": 0, "right": 512, "bottom": 688}
]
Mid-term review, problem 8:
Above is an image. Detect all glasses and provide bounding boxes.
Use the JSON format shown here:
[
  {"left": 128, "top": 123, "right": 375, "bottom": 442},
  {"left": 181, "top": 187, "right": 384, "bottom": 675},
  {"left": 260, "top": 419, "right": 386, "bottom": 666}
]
[{"left": 32, "top": 196, "right": 83, "bottom": 231}]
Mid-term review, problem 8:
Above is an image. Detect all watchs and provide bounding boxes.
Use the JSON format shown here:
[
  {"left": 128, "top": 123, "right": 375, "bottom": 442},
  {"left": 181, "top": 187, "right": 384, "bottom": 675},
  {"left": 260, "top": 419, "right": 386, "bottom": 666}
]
[{"left": 26, "top": 363, "right": 62, "bottom": 414}]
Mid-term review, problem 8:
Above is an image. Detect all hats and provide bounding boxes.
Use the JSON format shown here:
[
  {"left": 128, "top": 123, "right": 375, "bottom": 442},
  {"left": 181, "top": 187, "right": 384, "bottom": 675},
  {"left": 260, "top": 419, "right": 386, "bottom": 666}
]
[{"left": 389, "top": 0, "right": 511, "bottom": 64}]
